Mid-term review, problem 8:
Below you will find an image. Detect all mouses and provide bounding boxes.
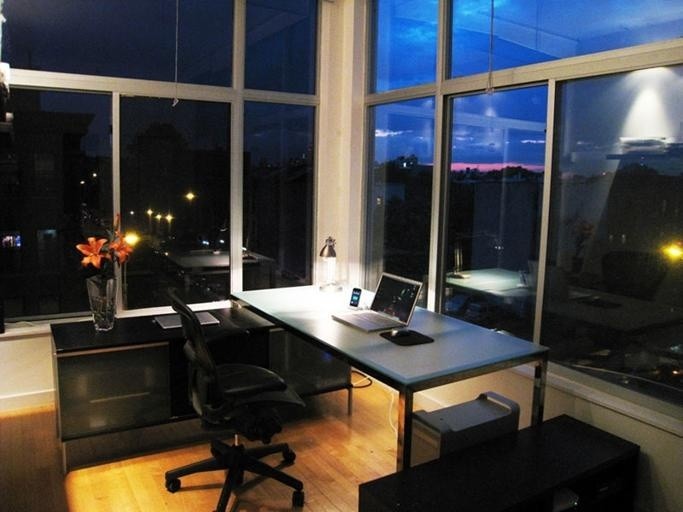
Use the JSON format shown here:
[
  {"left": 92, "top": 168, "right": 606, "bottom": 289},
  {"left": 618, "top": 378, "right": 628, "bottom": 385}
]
[{"left": 392, "top": 329, "right": 408, "bottom": 336}]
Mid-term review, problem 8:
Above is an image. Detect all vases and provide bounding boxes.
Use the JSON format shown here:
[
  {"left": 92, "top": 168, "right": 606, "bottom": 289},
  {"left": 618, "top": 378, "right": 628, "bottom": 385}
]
[{"left": 85, "top": 275, "right": 119, "bottom": 332}]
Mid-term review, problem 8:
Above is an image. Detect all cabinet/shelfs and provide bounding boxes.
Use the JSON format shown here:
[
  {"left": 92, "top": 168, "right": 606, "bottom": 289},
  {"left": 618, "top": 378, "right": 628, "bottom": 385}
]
[
  {"left": 359, "top": 413, "right": 641, "bottom": 512},
  {"left": 50, "top": 307, "right": 352, "bottom": 475}
]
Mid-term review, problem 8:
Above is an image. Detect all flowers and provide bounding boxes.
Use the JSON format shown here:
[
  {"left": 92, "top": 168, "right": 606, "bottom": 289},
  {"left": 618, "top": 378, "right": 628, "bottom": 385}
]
[{"left": 75, "top": 211, "right": 133, "bottom": 297}]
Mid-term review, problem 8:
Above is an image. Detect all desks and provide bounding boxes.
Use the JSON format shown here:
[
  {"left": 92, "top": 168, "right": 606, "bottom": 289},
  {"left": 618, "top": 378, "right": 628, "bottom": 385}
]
[{"left": 229, "top": 282, "right": 551, "bottom": 471}]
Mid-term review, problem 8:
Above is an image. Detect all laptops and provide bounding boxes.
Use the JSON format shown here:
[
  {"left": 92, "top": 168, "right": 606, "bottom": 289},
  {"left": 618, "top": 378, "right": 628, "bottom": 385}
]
[
  {"left": 332, "top": 272, "right": 423, "bottom": 333},
  {"left": 526, "top": 259, "right": 592, "bottom": 300},
  {"left": 154, "top": 312, "right": 220, "bottom": 330}
]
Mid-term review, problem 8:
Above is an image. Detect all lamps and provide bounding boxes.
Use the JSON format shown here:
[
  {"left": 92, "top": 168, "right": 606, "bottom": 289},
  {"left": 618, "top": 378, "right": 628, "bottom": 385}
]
[{"left": 318, "top": 237, "right": 342, "bottom": 291}]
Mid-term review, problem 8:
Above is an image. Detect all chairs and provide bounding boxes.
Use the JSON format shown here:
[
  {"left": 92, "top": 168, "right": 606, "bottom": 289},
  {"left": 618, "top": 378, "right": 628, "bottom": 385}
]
[{"left": 165, "top": 288, "right": 306, "bottom": 512}]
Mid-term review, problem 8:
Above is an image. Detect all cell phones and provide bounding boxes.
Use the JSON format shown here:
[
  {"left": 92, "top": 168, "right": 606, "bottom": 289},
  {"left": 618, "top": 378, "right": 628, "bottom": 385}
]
[{"left": 350, "top": 287, "right": 361, "bottom": 307}]
